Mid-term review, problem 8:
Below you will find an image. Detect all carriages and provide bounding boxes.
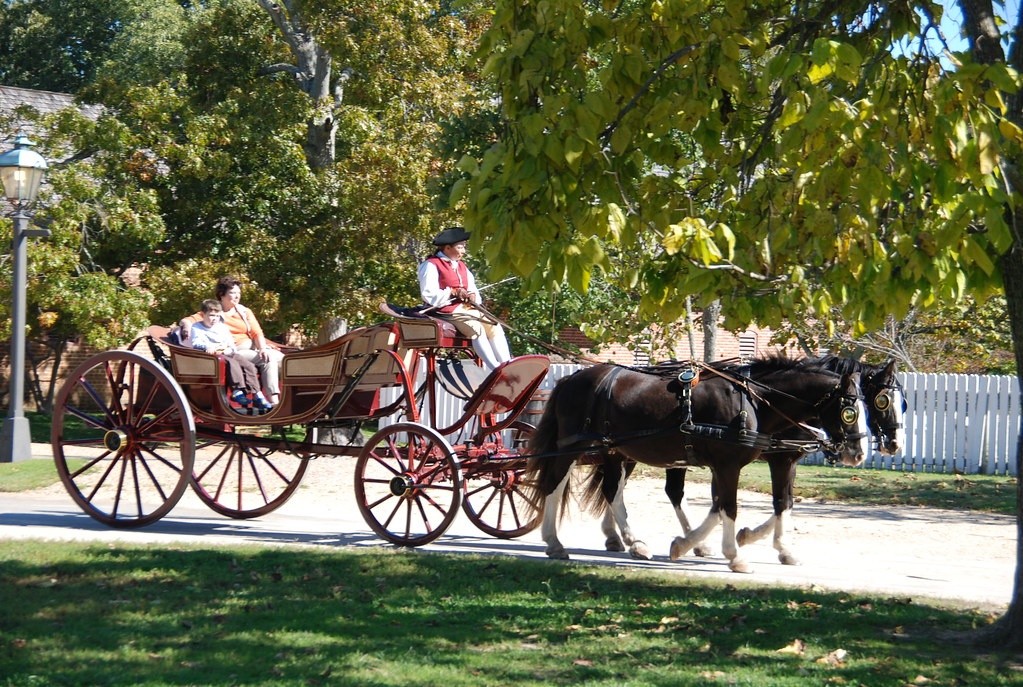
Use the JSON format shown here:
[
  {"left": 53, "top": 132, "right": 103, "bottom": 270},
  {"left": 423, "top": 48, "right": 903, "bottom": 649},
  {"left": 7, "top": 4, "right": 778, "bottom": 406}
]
[{"left": 53, "top": 303, "right": 910, "bottom": 574}]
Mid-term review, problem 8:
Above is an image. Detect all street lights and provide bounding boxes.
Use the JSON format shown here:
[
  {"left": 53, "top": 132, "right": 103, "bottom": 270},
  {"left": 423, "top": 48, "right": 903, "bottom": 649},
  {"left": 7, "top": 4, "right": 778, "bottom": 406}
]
[{"left": 0, "top": 128, "right": 49, "bottom": 464}]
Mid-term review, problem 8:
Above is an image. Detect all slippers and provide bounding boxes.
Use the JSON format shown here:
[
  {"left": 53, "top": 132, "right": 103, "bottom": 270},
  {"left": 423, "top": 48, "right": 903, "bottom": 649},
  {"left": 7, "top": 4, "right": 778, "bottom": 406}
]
[
  {"left": 230, "top": 391, "right": 251, "bottom": 404},
  {"left": 253, "top": 395, "right": 271, "bottom": 409}
]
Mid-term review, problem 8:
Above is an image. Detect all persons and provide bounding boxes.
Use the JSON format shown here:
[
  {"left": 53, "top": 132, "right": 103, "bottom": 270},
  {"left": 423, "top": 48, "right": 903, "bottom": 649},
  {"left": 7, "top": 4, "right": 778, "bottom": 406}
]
[
  {"left": 191, "top": 299, "right": 271, "bottom": 409},
  {"left": 180, "top": 276, "right": 285, "bottom": 404},
  {"left": 418, "top": 227, "right": 511, "bottom": 371}
]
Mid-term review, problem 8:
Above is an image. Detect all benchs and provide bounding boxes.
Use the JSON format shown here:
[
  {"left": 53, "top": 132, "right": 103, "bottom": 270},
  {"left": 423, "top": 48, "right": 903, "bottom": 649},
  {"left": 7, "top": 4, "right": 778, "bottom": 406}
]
[
  {"left": 159, "top": 335, "right": 281, "bottom": 383},
  {"left": 379, "top": 300, "right": 472, "bottom": 348}
]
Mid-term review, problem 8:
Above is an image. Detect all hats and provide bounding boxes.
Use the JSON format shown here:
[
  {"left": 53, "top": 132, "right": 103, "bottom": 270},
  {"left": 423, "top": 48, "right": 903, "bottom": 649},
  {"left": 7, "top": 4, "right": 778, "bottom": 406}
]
[{"left": 432, "top": 227, "right": 472, "bottom": 246}]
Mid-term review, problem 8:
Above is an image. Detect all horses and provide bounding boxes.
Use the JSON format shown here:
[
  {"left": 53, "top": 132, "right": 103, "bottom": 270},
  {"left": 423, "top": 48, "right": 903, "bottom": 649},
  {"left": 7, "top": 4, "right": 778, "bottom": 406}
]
[
  {"left": 577, "top": 353, "right": 908, "bottom": 566},
  {"left": 518, "top": 345, "right": 868, "bottom": 573}
]
